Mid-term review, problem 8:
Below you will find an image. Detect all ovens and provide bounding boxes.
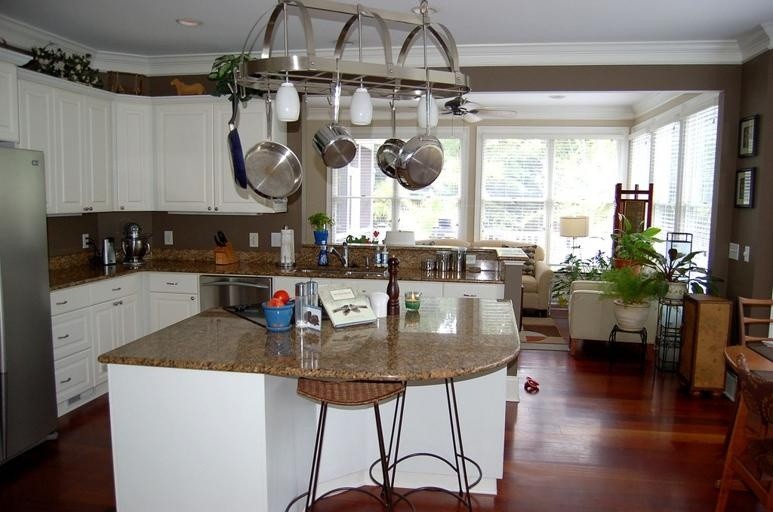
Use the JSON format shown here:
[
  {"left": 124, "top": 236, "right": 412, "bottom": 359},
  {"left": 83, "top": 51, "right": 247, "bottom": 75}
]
[{"left": 197, "top": 274, "right": 272, "bottom": 313}]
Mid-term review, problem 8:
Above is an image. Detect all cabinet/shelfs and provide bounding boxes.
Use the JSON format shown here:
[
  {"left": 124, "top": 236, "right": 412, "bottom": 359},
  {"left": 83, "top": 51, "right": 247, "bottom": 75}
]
[
  {"left": 149, "top": 92, "right": 280, "bottom": 217},
  {"left": 47, "top": 273, "right": 142, "bottom": 412},
  {"left": 149, "top": 273, "right": 195, "bottom": 333},
  {"left": 674, "top": 290, "right": 731, "bottom": 401},
  {"left": 7, "top": 67, "right": 114, "bottom": 214},
  {"left": 113, "top": 93, "right": 153, "bottom": 212}
]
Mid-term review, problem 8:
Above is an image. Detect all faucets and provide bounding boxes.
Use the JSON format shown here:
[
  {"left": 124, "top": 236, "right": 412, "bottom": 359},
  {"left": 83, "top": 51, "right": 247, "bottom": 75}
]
[{"left": 328, "top": 241, "right": 349, "bottom": 269}]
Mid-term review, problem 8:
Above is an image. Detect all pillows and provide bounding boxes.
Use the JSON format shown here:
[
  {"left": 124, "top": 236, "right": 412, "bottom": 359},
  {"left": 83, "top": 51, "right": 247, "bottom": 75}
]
[{"left": 500, "top": 245, "right": 535, "bottom": 276}]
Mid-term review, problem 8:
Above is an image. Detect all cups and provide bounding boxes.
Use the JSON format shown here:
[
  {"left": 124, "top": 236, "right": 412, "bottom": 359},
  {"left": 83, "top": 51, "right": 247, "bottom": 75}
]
[
  {"left": 405, "top": 289, "right": 422, "bottom": 312},
  {"left": 369, "top": 292, "right": 389, "bottom": 317}
]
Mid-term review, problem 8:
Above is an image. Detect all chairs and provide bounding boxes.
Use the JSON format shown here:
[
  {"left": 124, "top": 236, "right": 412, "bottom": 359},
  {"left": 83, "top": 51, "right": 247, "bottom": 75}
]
[
  {"left": 734, "top": 295, "right": 773, "bottom": 347},
  {"left": 569, "top": 273, "right": 660, "bottom": 361}
]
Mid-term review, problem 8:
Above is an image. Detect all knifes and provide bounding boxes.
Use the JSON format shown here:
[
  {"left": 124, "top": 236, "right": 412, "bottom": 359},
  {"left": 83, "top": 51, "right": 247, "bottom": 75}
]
[{"left": 213, "top": 230, "right": 227, "bottom": 247}]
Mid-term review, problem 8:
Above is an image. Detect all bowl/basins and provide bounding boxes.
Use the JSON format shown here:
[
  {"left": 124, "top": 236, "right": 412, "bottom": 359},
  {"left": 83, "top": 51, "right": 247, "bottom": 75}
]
[{"left": 260, "top": 302, "right": 295, "bottom": 327}]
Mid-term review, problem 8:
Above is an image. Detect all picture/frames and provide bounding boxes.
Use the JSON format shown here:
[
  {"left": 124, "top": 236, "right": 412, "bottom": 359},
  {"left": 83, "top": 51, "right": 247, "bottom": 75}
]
[
  {"left": 738, "top": 113, "right": 757, "bottom": 158},
  {"left": 733, "top": 167, "right": 755, "bottom": 209}
]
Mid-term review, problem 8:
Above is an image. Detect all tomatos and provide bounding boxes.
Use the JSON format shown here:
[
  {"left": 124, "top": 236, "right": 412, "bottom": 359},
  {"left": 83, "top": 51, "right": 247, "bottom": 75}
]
[
  {"left": 269, "top": 297, "right": 284, "bottom": 308},
  {"left": 275, "top": 290, "right": 289, "bottom": 304}
]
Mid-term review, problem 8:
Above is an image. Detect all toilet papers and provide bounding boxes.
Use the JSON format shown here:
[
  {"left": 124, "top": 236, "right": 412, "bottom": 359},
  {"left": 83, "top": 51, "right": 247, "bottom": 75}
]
[
  {"left": 370, "top": 291, "right": 390, "bottom": 318},
  {"left": 280, "top": 228, "right": 295, "bottom": 265}
]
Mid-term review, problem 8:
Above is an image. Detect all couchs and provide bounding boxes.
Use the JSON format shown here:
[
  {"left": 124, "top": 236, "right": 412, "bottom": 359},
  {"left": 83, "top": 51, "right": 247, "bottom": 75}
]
[{"left": 415, "top": 237, "right": 553, "bottom": 316}]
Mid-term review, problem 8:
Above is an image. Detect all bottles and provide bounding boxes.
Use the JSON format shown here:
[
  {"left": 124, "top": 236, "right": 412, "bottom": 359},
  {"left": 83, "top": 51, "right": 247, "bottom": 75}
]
[
  {"left": 280, "top": 223, "right": 296, "bottom": 267},
  {"left": 294, "top": 281, "right": 317, "bottom": 328},
  {"left": 435, "top": 250, "right": 451, "bottom": 272},
  {"left": 422, "top": 259, "right": 435, "bottom": 271},
  {"left": 450, "top": 247, "right": 466, "bottom": 273},
  {"left": 102, "top": 236, "right": 117, "bottom": 265},
  {"left": 318, "top": 240, "right": 327, "bottom": 266},
  {"left": 374, "top": 243, "right": 388, "bottom": 267},
  {"left": 421, "top": 271, "right": 466, "bottom": 282}
]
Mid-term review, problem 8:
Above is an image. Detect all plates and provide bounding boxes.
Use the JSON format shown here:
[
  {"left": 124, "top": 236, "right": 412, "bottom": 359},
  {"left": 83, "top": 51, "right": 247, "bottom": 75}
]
[{"left": 265, "top": 323, "right": 291, "bottom": 332}]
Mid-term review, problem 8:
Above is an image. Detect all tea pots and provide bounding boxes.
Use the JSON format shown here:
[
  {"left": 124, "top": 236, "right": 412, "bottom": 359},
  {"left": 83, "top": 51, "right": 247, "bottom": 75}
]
[{"left": 121, "top": 222, "right": 150, "bottom": 265}]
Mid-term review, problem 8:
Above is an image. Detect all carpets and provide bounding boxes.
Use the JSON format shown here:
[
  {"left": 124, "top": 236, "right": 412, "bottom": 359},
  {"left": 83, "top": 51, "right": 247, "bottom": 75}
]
[{"left": 518, "top": 316, "right": 569, "bottom": 349}]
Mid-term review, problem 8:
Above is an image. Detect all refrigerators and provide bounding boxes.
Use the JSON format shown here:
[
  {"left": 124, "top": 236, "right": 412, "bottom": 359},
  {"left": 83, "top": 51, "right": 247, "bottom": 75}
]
[{"left": 1, "top": 145, "right": 60, "bottom": 466}]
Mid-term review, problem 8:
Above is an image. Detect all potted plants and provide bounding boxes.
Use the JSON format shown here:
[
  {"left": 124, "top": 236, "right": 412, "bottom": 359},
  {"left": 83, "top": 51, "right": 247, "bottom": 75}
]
[
  {"left": 598, "top": 264, "right": 674, "bottom": 332},
  {"left": 306, "top": 209, "right": 332, "bottom": 244},
  {"left": 652, "top": 248, "right": 724, "bottom": 302},
  {"left": 609, "top": 213, "right": 666, "bottom": 276}
]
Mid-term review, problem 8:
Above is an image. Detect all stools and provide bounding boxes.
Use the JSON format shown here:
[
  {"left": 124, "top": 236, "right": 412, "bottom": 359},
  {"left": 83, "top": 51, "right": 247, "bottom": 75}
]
[
  {"left": 284, "top": 375, "right": 406, "bottom": 512},
  {"left": 367, "top": 378, "right": 483, "bottom": 512}
]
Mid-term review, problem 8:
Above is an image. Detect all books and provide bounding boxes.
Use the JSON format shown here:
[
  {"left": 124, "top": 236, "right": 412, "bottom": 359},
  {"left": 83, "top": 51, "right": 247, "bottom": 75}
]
[{"left": 316, "top": 284, "right": 378, "bottom": 328}]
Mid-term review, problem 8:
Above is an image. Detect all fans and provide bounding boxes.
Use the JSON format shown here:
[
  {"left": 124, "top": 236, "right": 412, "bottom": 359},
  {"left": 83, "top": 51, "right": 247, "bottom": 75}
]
[{"left": 407, "top": 96, "right": 515, "bottom": 123}]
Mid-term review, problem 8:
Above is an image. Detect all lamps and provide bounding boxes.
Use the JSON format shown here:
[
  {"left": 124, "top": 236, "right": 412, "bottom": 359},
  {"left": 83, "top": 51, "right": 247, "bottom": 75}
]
[
  {"left": 230, "top": 0, "right": 469, "bottom": 129},
  {"left": 558, "top": 216, "right": 589, "bottom": 258}
]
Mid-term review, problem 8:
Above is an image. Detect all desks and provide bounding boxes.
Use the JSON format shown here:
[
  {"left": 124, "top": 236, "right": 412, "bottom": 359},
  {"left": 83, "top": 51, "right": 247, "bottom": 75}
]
[
  {"left": 715, "top": 338, "right": 773, "bottom": 512},
  {"left": 605, "top": 327, "right": 651, "bottom": 377},
  {"left": 655, "top": 299, "right": 685, "bottom": 372}
]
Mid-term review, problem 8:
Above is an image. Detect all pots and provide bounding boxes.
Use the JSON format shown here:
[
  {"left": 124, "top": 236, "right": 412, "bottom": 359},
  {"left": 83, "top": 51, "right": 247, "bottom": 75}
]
[
  {"left": 394, "top": 92, "right": 444, "bottom": 191},
  {"left": 223, "top": 75, "right": 246, "bottom": 190},
  {"left": 376, "top": 106, "right": 405, "bottom": 179},
  {"left": 241, "top": 99, "right": 302, "bottom": 199},
  {"left": 308, "top": 81, "right": 357, "bottom": 171}
]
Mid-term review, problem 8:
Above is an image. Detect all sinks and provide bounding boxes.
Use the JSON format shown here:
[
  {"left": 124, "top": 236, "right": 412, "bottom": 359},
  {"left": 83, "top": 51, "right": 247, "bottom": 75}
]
[{"left": 302, "top": 269, "right": 385, "bottom": 275}]
